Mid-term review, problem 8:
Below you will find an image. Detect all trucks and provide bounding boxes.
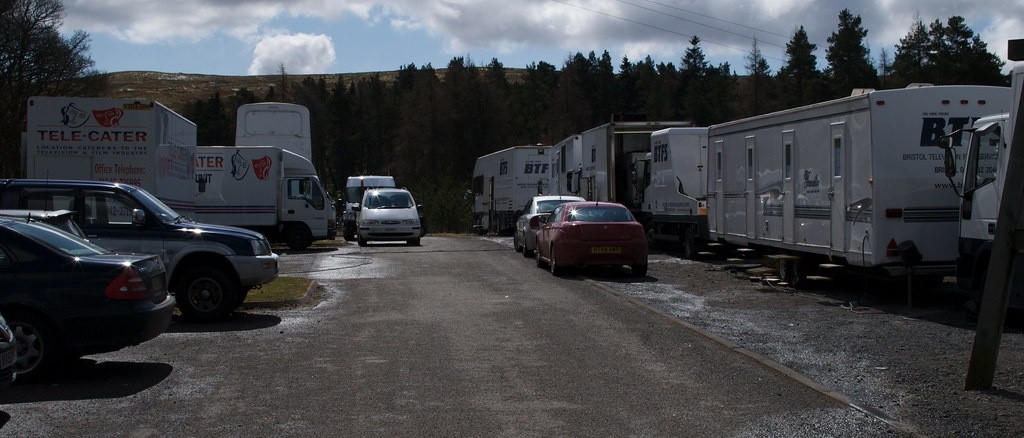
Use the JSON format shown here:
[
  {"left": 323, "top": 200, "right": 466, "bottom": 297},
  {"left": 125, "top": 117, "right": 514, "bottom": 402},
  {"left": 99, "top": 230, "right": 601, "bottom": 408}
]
[
  {"left": 565, "top": 114, "right": 696, "bottom": 227},
  {"left": 933, "top": 67, "right": 1024, "bottom": 320},
  {"left": 196, "top": 145, "right": 338, "bottom": 252},
  {"left": 464, "top": 144, "right": 555, "bottom": 237},
  {"left": 627, "top": 82, "right": 1014, "bottom": 300},
  {"left": 537, "top": 131, "right": 582, "bottom": 202}
]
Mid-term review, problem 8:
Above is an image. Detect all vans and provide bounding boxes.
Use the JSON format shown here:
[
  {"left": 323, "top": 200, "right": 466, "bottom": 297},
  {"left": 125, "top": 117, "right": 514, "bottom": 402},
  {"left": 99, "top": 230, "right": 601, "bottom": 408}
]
[{"left": 338, "top": 175, "right": 397, "bottom": 242}]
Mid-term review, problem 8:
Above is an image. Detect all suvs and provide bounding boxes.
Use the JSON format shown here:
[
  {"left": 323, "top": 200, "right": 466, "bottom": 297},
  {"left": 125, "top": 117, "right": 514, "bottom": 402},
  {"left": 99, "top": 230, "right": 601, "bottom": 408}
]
[
  {"left": 351, "top": 185, "right": 425, "bottom": 247},
  {"left": 0, "top": 177, "right": 281, "bottom": 325}
]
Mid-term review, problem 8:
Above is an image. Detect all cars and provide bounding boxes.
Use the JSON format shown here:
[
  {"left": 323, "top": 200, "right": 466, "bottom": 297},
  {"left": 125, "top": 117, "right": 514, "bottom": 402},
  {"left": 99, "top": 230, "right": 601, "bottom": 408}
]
[
  {"left": 512, "top": 196, "right": 584, "bottom": 258},
  {"left": 532, "top": 201, "right": 648, "bottom": 277},
  {"left": 0, "top": 209, "right": 88, "bottom": 241},
  {"left": 0, "top": 315, "right": 20, "bottom": 390},
  {"left": 0, "top": 219, "right": 176, "bottom": 387}
]
